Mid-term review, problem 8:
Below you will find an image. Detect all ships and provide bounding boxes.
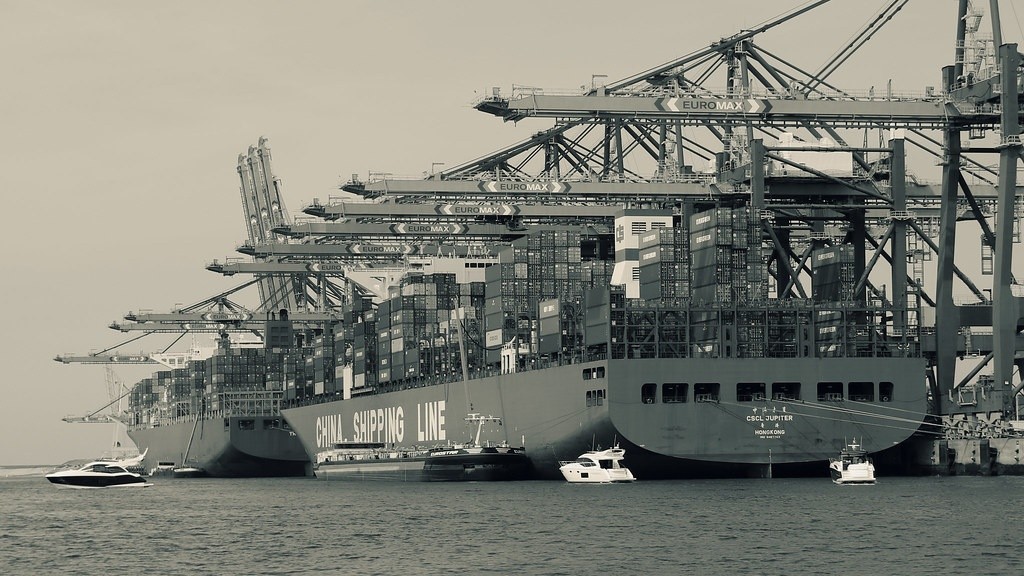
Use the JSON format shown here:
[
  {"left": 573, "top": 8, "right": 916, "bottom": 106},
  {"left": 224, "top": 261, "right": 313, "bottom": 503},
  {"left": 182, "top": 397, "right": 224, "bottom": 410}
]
[{"left": 52, "top": 0, "right": 1024, "bottom": 485}]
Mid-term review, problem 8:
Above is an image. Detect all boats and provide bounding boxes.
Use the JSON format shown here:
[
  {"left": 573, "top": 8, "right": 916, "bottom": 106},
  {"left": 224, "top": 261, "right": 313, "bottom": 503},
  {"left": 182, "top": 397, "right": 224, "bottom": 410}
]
[
  {"left": 312, "top": 415, "right": 534, "bottom": 482},
  {"left": 826, "top": 436, "right": 880, "bottom": 486},
  {"left": 44, "top": 462, "right": 153, "bottom": 490},
  {"left": 561, "top": 445, "right": 637, "bottom": 485}
]
